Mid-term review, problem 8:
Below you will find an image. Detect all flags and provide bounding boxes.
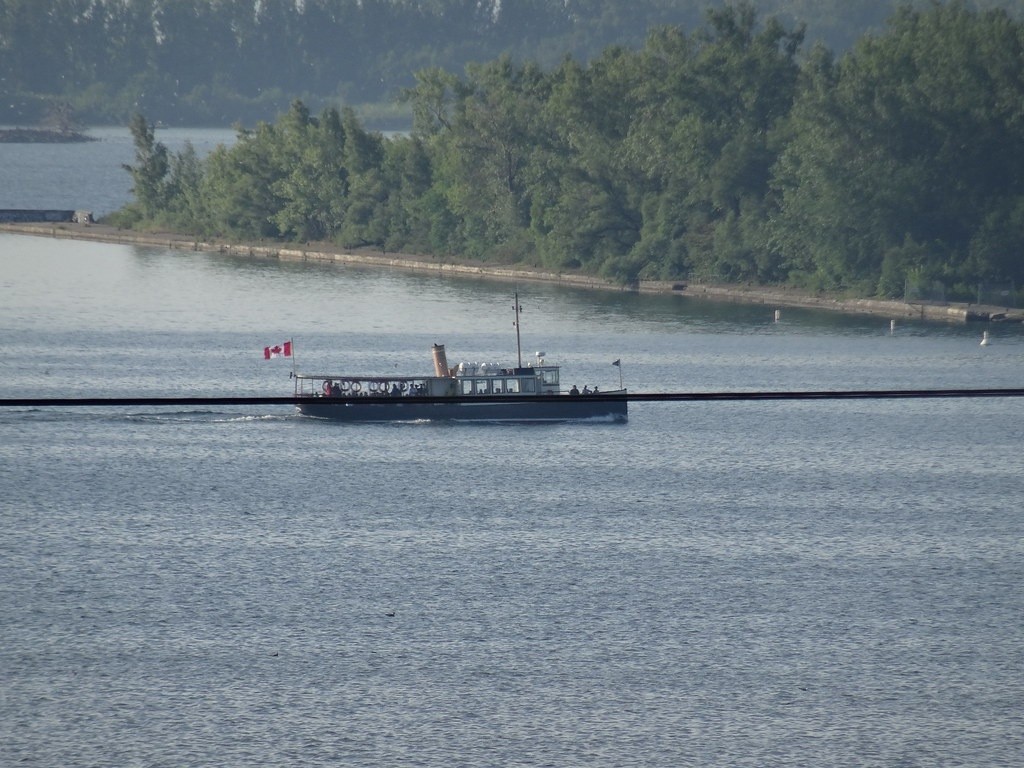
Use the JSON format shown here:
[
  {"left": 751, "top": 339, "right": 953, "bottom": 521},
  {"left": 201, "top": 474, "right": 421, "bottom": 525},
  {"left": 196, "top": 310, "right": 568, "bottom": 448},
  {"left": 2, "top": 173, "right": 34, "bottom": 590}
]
[{"left": 264, "top": 342, "right": 292, "bottom": 359}]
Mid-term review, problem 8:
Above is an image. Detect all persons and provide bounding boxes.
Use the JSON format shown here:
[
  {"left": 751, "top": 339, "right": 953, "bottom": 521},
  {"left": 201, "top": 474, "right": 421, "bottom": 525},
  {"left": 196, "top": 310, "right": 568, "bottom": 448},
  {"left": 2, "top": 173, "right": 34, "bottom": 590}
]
[
  {"left": 479, "top": 388, "right": 513, "bottom": 393},
  {"left": 327, "top": 382, "right": 341, "bottom": 395},
  {"left": 570, "top": 385, "right": 579, "bottom": 394},
  {"left": 392, "top": 384, "right": 406, "bottom": 396},
  {"left": 594, "top": 386, "right": 599, "bottom": 392},
  {"left": 409, "top": 383, "right": 426, "bottom": 396},
  {"left": 582, "top": 385, "right": 592, "bottom": 394}
]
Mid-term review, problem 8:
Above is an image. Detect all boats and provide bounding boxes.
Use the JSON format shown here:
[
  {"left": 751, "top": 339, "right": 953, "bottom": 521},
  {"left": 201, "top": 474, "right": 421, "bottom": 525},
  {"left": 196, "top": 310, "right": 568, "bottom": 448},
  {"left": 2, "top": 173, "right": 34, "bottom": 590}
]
[{"left": 289, "top": 290, "right": 629, "bottom": 422}]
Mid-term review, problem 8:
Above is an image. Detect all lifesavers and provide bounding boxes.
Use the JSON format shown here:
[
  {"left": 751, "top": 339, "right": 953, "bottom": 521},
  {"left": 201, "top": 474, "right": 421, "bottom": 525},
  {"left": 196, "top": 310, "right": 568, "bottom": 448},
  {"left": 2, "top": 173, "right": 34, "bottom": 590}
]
[
  {"left": 398, "top": 381, "right": 409, "bottom": 392},
  {"left": 368, "top": 381, "right": 379, "bottom": 392},
  {"left": 379, "top": 382, "right": 390, "bottom": 392},
  {"left": 322, "top": 381, "right": 328, "bottom": 392},
  {"left": 340, "top": 381, "right": 351, "bottom": 391},
  {"left": 351, "top": 381, "right": 362, "bottom": 392}
]
